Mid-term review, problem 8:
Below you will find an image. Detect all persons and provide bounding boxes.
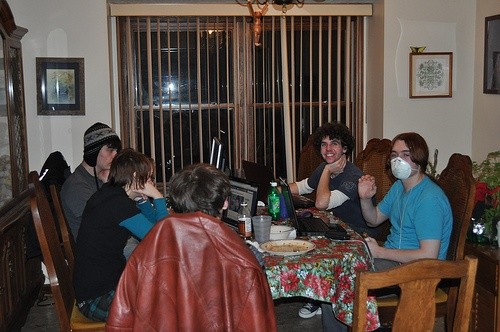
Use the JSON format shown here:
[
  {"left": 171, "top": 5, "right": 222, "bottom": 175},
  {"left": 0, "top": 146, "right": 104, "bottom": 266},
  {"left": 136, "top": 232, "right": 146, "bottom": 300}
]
[
  {"left": 322, "top": 129, "right": 453, "bottom": 332},
  {"left": 276, "top": 120, "right": 376, "bottom": 318},
  {"left": 72, "top": 150, "right": 170, "bottom": 323},
  {"left": 58, "top": 122, "right": 123, "bottom": 249},
  {"left": 111, "top": 164, "right": 279, "bottom": 332}
]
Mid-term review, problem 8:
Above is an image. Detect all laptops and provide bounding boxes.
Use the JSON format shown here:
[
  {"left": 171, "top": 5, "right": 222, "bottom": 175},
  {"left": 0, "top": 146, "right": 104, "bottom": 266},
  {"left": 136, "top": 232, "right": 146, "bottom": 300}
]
[
  {"left": 221, "top": 175, "right": 259, "bottom": 232},
  {"left": 279, "top": 176, "right": 336, "bottom": 239}
]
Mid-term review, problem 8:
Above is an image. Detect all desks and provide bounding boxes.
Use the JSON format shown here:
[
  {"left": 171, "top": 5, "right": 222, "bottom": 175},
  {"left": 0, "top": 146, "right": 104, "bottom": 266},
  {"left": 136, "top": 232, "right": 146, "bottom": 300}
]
[{"left": 257, "top": 196, "right": 381, "bottom": 331}]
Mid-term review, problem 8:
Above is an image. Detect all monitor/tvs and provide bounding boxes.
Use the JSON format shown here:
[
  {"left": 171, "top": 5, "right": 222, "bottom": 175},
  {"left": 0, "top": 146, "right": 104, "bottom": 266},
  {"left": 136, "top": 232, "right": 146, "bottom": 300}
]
[
  {"left": 209, "top": 136, "right": 225, "bottom": 168},
  {"left": 240, "top": 158, "right": 272, "bottom": 205}
]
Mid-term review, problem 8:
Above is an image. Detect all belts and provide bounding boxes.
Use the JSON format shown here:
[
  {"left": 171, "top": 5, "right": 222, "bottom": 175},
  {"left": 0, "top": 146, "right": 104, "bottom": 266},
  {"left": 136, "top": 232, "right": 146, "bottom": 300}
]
[{"left": 75, "top": 300, "right": 86, "bottom": 311}]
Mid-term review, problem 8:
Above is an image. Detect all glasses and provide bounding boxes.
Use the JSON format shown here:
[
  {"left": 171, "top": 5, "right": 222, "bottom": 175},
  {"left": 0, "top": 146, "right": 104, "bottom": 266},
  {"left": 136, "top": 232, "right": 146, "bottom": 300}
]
[{"left": 147, "top": 174, "right": 156, "bottom": 182}]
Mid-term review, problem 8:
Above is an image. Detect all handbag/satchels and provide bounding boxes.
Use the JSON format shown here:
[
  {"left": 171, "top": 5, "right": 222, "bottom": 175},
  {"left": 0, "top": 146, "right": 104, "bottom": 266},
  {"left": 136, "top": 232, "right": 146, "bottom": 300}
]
[{"left": 465, "top": 194, "right": 494, "bottom": 245}]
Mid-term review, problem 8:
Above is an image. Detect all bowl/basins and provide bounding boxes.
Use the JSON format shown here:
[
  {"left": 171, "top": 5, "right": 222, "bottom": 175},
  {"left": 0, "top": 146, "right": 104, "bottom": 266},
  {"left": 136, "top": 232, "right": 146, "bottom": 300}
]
[{"left": 270, "top": 225, "right": 294, "bottom": 241}]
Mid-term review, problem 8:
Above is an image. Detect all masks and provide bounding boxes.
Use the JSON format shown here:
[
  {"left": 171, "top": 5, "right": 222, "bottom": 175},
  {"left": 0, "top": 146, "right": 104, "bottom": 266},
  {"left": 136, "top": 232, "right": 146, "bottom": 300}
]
[{"left": 390, "top": 157, "right": 422, "bottom": 180}]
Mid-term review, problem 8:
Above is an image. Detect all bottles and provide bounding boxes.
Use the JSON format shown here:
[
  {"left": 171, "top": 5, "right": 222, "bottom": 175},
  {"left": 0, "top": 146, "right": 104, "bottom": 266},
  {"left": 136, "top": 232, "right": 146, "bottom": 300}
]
[
  {"left": 268, "top": 181, "right": 281, "bottom": 221},
  {"left": 238, "top": 203, "right": 252, "bottom": 240}
]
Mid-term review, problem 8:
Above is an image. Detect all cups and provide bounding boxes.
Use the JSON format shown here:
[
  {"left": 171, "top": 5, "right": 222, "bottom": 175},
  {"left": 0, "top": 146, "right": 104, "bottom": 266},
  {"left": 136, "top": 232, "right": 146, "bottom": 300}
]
[{"left": 251, "top": 216, "right": 272, "bottom": 245}]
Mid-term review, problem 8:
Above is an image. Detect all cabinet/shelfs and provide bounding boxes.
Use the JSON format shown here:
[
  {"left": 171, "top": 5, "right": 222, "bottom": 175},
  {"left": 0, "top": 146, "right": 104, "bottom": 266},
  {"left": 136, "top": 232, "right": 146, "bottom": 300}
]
[{"left": 464, "top": 244, "right": 500, "bottom": 332}]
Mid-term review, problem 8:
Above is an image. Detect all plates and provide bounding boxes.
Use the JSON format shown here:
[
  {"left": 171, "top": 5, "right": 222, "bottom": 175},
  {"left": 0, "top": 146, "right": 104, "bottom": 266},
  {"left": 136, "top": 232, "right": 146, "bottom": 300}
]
[{"left": 259, "top": 239, "right": 316, "bottom": 255}]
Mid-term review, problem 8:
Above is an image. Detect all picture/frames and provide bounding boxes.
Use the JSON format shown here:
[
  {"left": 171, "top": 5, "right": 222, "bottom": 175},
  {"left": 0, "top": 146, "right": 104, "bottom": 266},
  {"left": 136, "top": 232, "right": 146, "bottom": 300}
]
[
  {"left": 482, "top": 14, "right": 500, "bottom": 94},
  {"left": 409, "top": 52, "right": 453, "bottom": 98},
  {"left": 36, "top": 57, "right": 85, "bottom": 115}
]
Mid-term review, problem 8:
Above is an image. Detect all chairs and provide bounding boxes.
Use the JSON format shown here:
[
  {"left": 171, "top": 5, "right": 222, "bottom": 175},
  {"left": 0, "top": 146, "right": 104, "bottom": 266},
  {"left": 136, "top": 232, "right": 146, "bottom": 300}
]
[
  {"left": 298, "top": 134, "right": 477, "bottom": 331},
  {"left": 48, "top": 184, "right": 75, "bottom": 271},
  {"left": 27, "top": 171, "right": 106, "bottom": 332},
  {"left": 353, "top": 254, "right": 479, "bottom": 332}
]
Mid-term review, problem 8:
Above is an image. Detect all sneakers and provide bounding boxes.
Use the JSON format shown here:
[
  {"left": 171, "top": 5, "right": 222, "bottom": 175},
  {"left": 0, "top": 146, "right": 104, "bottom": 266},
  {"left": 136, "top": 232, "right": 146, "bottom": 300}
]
[{"left": 298, "top": 299, "right": 321, "bottom": 318}]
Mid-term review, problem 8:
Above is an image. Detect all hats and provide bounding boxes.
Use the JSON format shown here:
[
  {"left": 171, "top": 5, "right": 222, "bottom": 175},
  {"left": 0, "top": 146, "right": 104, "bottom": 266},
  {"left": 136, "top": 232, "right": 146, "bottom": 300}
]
[{"left": 83, "top": 123, "right": 121, "bottom": 190}]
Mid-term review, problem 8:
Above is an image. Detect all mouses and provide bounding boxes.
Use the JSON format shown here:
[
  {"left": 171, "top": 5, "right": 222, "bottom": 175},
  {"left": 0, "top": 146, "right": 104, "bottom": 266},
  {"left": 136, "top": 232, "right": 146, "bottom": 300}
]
[{"left": 303, "top": 211, "right": 312, "bottom": 218}]
